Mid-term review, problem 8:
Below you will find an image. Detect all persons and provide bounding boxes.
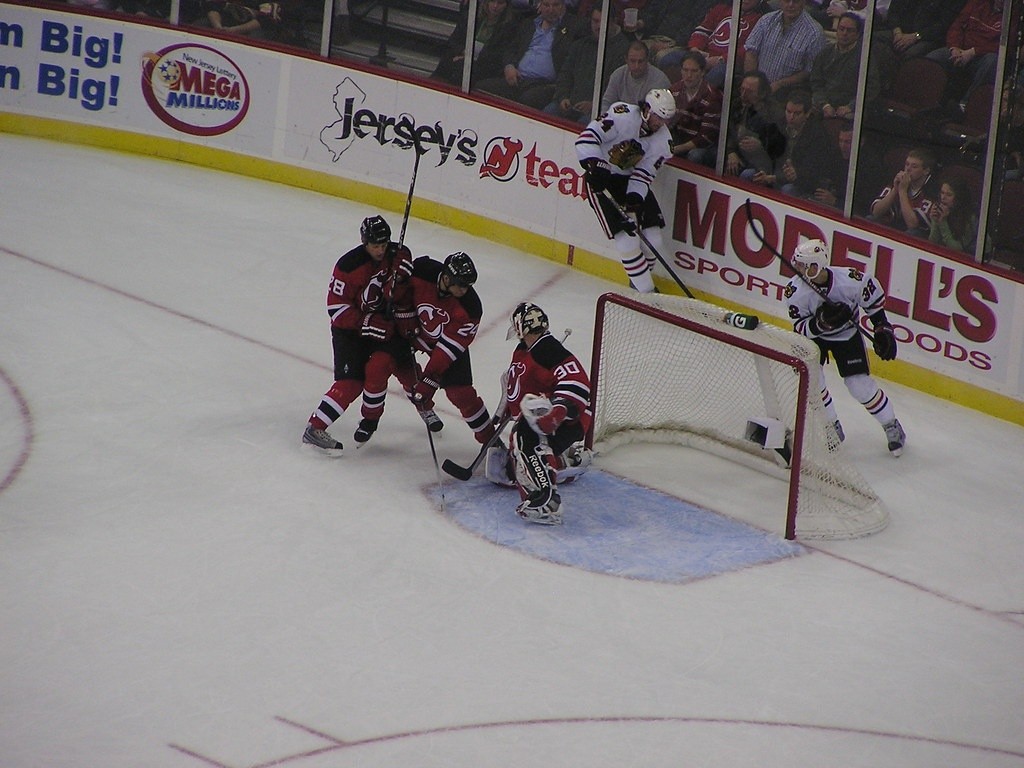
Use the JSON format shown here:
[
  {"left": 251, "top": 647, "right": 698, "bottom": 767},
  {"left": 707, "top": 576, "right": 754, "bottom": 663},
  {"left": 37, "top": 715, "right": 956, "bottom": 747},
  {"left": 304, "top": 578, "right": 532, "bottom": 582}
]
[
  {"left": 498, "top": 302, "right": 592, "bottom": 518},
  {"left": 426, "top": 0, "right": 1024, "bottom": 259},
  {"left": 782, "top": 239, "right": 906, "bottom": 451},
  {"left": 574, "top": 88, "right": 677, "bottom": 294},
  {"left": 302, "top": 215, "right": 508, "bottom": 450},
  {"left": 69, "top": 0, "right": 358, "bottom": 46}
]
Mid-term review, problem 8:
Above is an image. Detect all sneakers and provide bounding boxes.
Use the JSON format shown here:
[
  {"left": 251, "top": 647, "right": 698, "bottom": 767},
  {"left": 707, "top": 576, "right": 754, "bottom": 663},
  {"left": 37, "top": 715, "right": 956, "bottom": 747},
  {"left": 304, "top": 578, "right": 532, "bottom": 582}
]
[
  {"left": 300, "top": 423, "right": 344, "bottom": 456},
  {"left": 826, "top": 420, "right": 845, "bottom": 451},
  {"left": 883, "top": 421, "right": 906, "bottom": 458},
  {"left": 354, "top": 416, "right": 378, "bottom": 450},
  {"left": 417, "top": 410, "right": 443, "bottom": 439}
]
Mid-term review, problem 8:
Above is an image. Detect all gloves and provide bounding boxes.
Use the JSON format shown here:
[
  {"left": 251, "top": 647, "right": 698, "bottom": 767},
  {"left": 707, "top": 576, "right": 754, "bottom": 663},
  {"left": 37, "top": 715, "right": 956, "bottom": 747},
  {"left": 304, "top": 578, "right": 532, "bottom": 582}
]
[
  {"left": 873, "top": 321, "right": 898, "bottom": 362},
  {"left": 816, "top": 300, "right": 853, "bottom": 329},
  {"left": 534, "top": 402, "right": 566, "bottom": 435},
  {"left": 359, "top": 314, "right": 395, "bottom": 344},
  {"left": 589, "top": 157, "right": 612, "bottom": 194},
  {"left": 393, "top": 304, "right": 421, "bottom": 339},
  {"left": 619, "top": 198, "right": 644, "bottom": 238},
  {"left": 412, "top": 370, "right": 440, "bottom": 405}
]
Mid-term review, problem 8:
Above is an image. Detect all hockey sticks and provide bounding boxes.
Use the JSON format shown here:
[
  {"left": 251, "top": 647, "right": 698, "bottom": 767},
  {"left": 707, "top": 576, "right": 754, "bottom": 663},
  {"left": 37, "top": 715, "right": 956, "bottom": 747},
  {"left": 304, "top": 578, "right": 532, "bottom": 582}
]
[
  {"left": 384, "top": 115, "right": 422, "bottom": 318},
  {"left": 441, "top": 327, "right": 572, "bottom": 482},
  {"left": 409, "top": 337, "right": 445, "bottom": 513},
  {"left": 743, "top": 197, "right": 874, "bottom": 341},
  {"left": 604, "top": 188, "right": 694, "bottom": 299}
]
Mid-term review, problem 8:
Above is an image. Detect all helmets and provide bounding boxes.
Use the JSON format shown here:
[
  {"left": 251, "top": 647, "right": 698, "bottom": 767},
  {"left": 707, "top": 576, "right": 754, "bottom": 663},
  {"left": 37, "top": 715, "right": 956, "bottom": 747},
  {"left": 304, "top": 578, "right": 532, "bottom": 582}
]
[
  {"left": 510, "top": 302, "right": 549, "bottom": 336},
  {"left": 445, "top": 252, "right": 478, "bottom": 287},
  {"left": 645, "top": 87, "right": 677, "bottom": 121},
  {"left": 359, "top": 215, "right": 391, "bottom": 246},
  {"left": 792, "top": 238, "right": 830, "bottom": 270}
]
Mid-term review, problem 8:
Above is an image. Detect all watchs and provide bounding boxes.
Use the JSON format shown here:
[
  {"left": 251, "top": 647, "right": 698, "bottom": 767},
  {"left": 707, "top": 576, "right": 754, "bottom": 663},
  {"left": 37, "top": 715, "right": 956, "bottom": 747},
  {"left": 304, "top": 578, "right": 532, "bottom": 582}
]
[{"left": 913, "top": 32, "right": 921, "bottom": 42}]
